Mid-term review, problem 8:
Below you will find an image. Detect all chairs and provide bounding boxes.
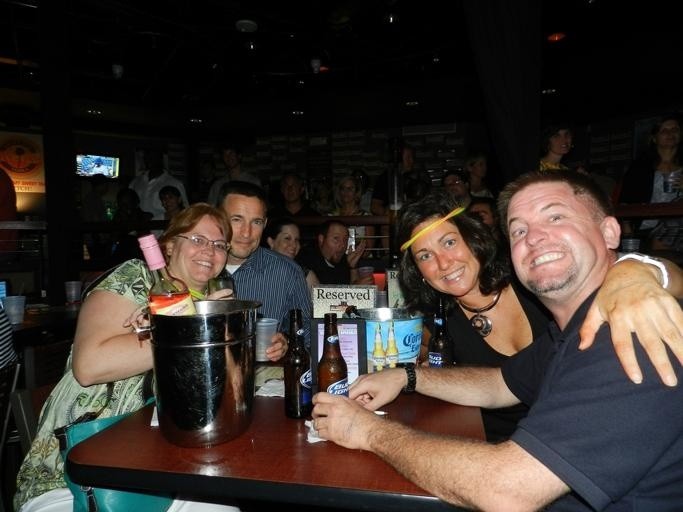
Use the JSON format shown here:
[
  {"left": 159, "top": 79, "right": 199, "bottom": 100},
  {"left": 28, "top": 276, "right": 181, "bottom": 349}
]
[{"left": 0, "top": 318, "right": 78, "bottom": 457}]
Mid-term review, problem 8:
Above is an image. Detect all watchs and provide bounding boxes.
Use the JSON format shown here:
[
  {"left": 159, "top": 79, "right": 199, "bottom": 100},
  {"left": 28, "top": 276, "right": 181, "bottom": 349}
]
[
  {"left": 613, "top": 252, "right": 669, "bottom": 289},
  {"left": 396, "top": 362, "right": 417, "bottom": 392}
]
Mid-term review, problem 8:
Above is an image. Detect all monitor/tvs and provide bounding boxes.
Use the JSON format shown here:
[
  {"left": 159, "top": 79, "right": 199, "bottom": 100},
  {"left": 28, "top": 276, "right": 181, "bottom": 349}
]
[{"left": 73, "top": 149, "right": 125, "bottom": 181}]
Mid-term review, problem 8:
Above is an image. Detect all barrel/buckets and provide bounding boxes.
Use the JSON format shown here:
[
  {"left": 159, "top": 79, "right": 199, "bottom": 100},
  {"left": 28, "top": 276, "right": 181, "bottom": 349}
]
[
  {"left": 344, "top": 307, "right": 425, "bottom": 375},
  {"left": 132, "top": 298, "right": 263, "bottom": 449}
]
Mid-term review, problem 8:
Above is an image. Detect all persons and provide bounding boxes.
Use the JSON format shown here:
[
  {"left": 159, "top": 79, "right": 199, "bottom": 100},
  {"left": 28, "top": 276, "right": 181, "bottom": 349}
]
[
  {"left": 100, "top": 142, "right": 504, "bottom": 289},
  {"left": 311, "top": 168, "right": 683, "bottom": 512},
  {"left": 215, "top": 180, "right": 310, "bottom": 361},
  {"left": 534, "top": 121, "right": 589, "bottom": 178},
  {"left": 398, "top": 195, "right": 682, "bottom": 385},
  {"left": 0, "top": 293, "right": 24, "bottom": 512},
  {"left": 12, "top": 202, "right": 241, "bottom": 512},
  {"left": 615, "top": 112, "right": 682, "bottom": 251}
]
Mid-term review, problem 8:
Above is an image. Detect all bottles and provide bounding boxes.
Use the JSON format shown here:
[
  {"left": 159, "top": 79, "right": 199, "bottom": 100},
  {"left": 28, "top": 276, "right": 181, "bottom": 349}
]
[
  {"left": 137, "top": 235, "right": 201, "bottom": 317},
  {"left": 372, "top": 324, "right": 385, "bottom": 371},
  {"left": 386, "top": 323, "right": 399, "bottom": 369},
  {"left": 318, "top": 312, "right": 349, "bottom": 399},
  {"left": 282, "top": 306, "right": 313, "bottom": 419},
  {"left": 427, "top": 295, "right": 455, "bottom": 368}
]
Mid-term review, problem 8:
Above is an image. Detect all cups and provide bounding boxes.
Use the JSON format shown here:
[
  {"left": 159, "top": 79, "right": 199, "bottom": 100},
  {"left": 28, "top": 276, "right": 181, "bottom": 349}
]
[
  {"left": 619, "top": 238, "right": 639, "bottom": 253},
  {"left": 1, "top": 295, "right": 24, "bottom": 323},
  {"left": 254, "top": 318, "right": 278, "bottom": 362},
  {"left": 356, "top": 266, "right": 374, "bottom": 282},
  {"left": 207, "top": 277, "right": 235, "bottom": 300},
  {"left": 63, "top": 280, "right": 82, "bottom": 301}
]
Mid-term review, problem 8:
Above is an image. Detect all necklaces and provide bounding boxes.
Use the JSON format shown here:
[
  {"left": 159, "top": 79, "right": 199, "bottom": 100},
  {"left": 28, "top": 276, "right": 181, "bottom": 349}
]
[{"left": 458, "top": 289, "right": 502, "bottom": 337}]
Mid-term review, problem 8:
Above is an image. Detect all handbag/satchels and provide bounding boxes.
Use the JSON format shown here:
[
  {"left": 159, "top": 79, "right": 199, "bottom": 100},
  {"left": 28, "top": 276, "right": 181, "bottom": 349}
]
[{"left": 50, "top": 375, "right": 173, "bottom": 512}]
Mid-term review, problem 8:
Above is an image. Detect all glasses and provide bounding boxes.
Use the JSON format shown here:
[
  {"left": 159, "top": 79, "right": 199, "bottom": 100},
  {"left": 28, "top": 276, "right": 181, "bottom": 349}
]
[{"left": 177, "top": 233, "right": 229, "bottom": 253}]
